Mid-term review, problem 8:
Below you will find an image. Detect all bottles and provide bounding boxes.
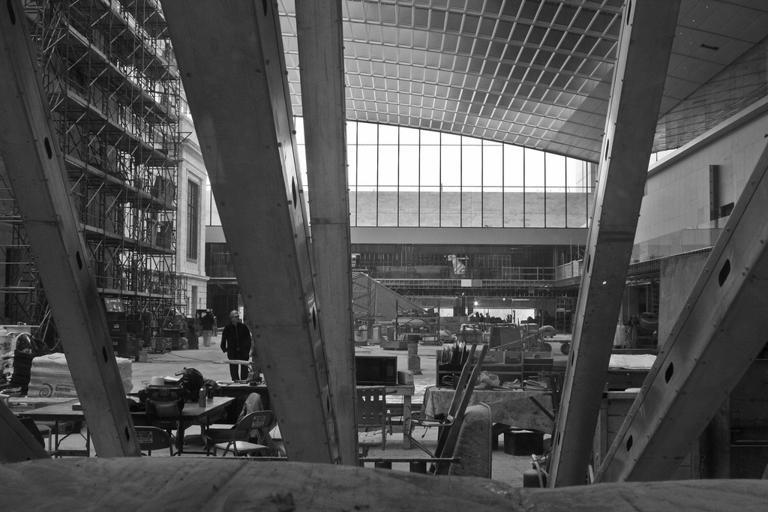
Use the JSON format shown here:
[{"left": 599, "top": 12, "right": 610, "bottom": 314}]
[{"left": 198, "top": 385, "right": 207, "bottom": 406}]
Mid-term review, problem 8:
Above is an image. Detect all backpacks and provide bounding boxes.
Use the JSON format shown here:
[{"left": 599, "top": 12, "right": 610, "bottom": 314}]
[{"left": 175, "top": 367, "right": 203, "bottom": 403}]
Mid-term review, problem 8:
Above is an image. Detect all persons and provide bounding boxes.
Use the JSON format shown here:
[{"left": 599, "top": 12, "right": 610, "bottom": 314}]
[
  {"left": 221, "top": 310, "right": 251, "bottom": 380},
  {"left": 202, "top": 308, "right": 213, "bottom": 346}
]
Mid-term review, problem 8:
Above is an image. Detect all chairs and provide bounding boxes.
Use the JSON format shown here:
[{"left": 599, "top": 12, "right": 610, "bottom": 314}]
[{"left": 17, "top": 355, "right": 564, "bottom": 457}]
